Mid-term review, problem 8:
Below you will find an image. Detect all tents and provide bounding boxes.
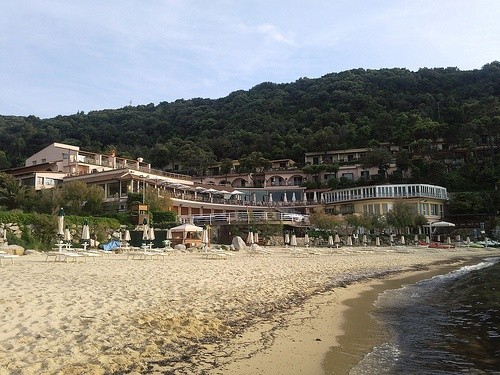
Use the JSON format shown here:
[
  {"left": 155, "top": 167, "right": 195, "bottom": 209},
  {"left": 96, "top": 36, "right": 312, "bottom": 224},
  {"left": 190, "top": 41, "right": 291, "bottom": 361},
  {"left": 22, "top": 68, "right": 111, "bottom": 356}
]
[
  {"left": 432, "top": 220, "right": 456, "bottom": 228},
  {"left": 170, "top": 224, "right": 204, "bottom": 240}
]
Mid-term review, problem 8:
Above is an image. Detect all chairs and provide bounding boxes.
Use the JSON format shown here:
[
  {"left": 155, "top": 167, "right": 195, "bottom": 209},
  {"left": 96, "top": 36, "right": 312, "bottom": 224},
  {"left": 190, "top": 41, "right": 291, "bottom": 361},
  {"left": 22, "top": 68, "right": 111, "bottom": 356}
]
[{"left": 0, "top": 239, "right": 418, "bottom": 266}]
